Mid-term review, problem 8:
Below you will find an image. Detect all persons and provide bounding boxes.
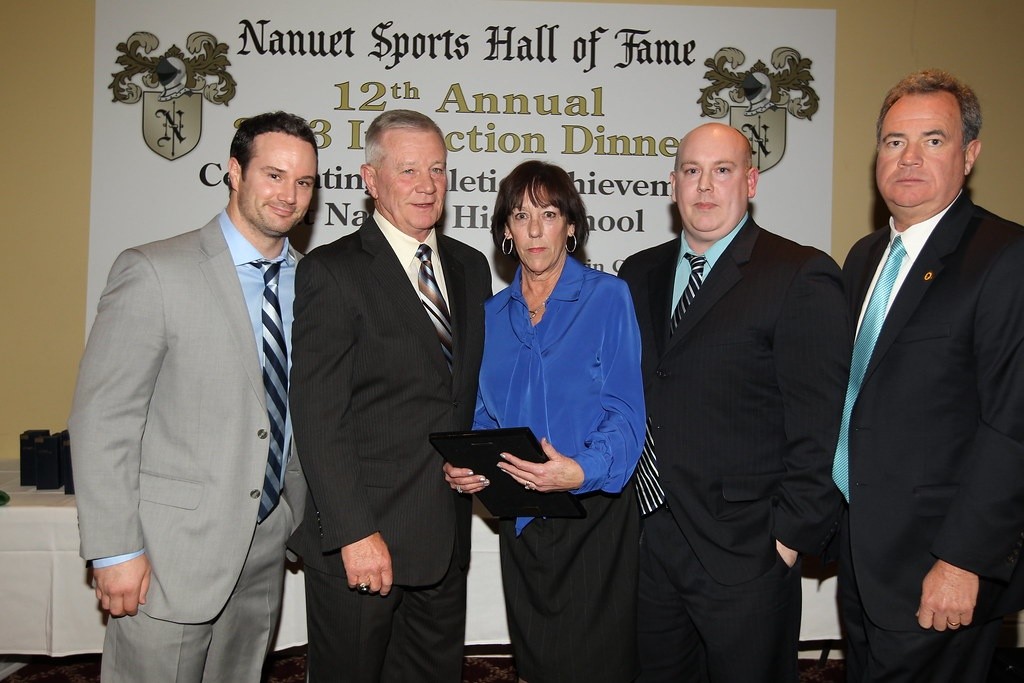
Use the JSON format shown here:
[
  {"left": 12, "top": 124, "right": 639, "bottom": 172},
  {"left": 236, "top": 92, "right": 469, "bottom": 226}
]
[
  {"left": 441, "top": 161, "right": 644, "bottom": 683},
  {"left": 67, "top": 111, "right": 318, "bottom": 683},
  {"left": 619, "top": 121, "right": 850, "bottom": 683},
  {"left": 824, "top": 67, "right": 1024, "bottom": 683},
  {"left": 289, "top": 110, "right": 494, "bottom": 683}
]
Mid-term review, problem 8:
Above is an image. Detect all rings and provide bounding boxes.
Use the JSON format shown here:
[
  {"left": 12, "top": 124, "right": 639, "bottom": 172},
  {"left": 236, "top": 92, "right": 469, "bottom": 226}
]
[
  {"left": 525, "top": 481, "right": 530, "bottom": 490},
  {"left": 357, "top": 583, "right": 370, "bottom": 592},
  {"left": 456, "top": 486, "right": 463, "bottom": 493},
  {"left": 947, "top": 620, "right": 960, "bottom": 626}
]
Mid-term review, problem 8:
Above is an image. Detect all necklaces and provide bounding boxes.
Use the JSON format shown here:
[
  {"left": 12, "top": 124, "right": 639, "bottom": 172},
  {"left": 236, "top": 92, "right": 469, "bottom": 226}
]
[{"left": 530, "top": 297, "right": 549, "bottom": 318}]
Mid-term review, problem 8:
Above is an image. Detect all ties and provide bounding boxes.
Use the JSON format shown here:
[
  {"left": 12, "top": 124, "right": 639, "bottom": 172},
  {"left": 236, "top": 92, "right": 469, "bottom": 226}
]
[
  {"left": 415, "top": 243, "right": 454, "bottom": 378},
  {"left": 831, "top": 234, "right": 904, "bottom": 506},
  {"left": 634, "top": 254, "right": 707, "bottom": 516},
  {"left": 246, "top": 259, "right": 289, "bottom": 524}
]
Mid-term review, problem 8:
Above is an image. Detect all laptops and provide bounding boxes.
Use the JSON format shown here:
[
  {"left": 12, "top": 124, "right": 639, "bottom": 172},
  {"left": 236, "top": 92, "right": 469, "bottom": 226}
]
[{"left": 429, "top": 426, "right": 588, "bottom": 519}]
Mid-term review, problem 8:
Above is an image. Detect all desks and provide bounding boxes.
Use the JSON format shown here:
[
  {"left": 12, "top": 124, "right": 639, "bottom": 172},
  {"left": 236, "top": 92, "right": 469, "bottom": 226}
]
[{"left": 0, "top": 464, "right": 841, "bottom": 659}]
